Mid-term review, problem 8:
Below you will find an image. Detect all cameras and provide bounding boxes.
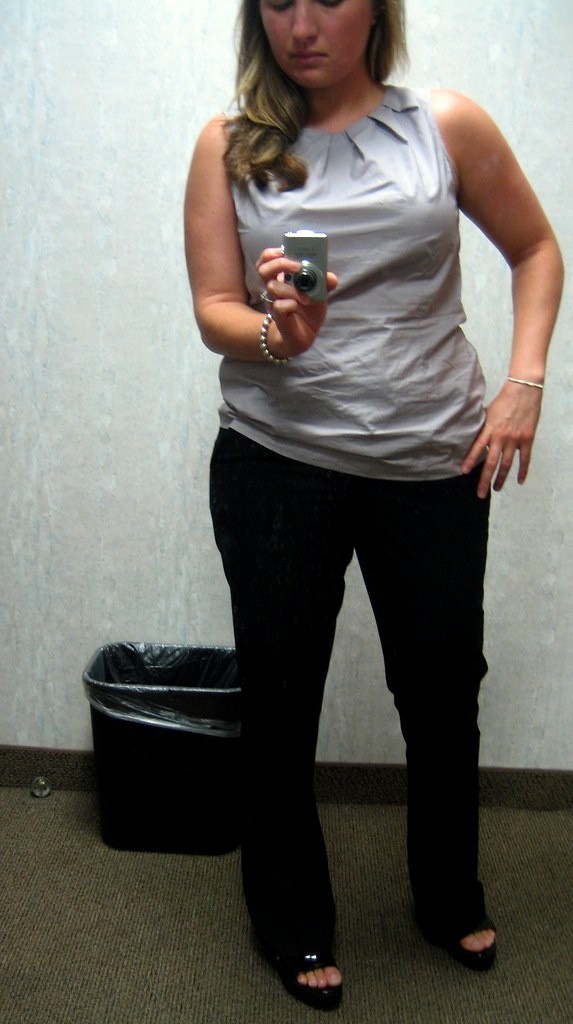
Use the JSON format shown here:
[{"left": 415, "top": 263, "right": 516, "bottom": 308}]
[{"left": 280, "top": 231, "right": 328, "bottom": 304}]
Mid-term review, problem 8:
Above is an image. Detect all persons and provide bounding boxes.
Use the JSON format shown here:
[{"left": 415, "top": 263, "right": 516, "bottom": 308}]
[{"left": 182, "top": 2, "right": 566, "bottom": 1012}]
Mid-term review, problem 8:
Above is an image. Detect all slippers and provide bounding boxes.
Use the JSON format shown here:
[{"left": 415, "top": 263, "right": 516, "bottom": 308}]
[
  {"left": 257, "top": 939, "right": 343, "bottom": 1012},
  {"left": 411, "top": 911, "right": 496, "bottom": 972}
]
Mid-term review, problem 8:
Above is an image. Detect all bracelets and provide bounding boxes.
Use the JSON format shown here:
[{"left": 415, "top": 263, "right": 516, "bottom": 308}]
[
  {"left": 257, "top": 314, "right": 295, "bottom": 366},
  {"left": 508, "top": 377, "right": 543, "bottom": 390}
]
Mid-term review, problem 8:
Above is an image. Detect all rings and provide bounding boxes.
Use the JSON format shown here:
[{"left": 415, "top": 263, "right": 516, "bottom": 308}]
[{"left": 258, "top": 290, "right": 274, "bottom": 304}]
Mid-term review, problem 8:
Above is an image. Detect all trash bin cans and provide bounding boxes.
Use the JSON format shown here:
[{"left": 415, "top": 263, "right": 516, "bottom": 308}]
[{"left": 84, "top": 638, "right": 247, "bottom": 860}]
